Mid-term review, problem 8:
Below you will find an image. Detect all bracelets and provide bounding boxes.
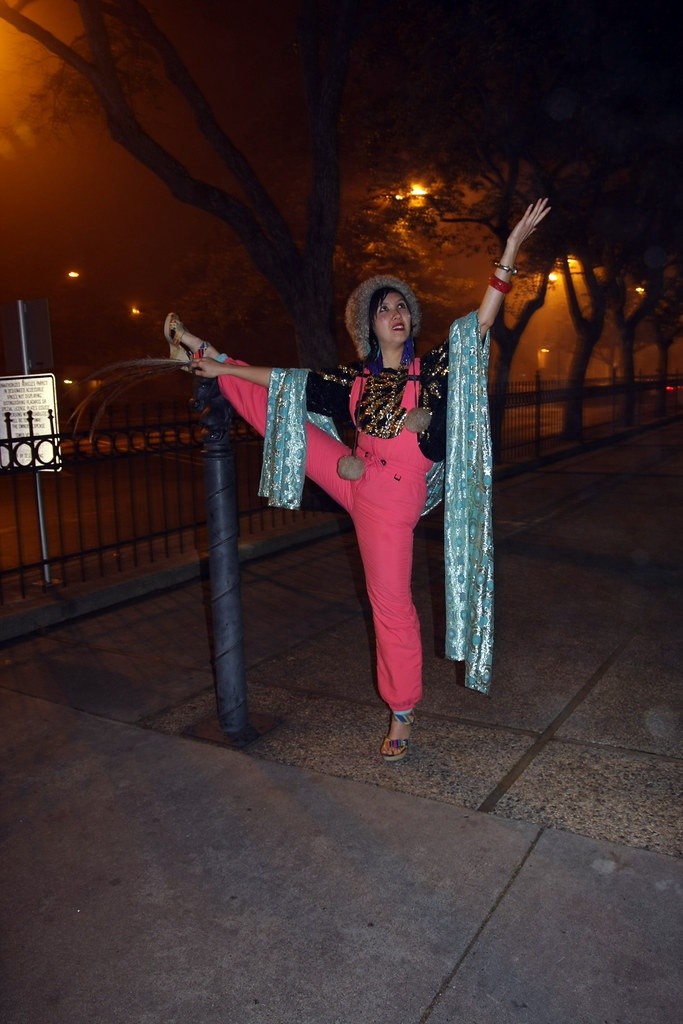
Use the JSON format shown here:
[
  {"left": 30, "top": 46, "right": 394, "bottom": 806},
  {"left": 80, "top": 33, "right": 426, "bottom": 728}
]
[
  {"left": 488, "top": 274, "right": 512, "bottom": 294},
  {"left": 494, "top": 262, "right": 517, "bottom": 275}
]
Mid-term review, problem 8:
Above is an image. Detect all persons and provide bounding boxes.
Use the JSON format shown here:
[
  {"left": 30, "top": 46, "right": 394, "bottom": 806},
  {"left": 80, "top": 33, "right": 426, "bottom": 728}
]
[{"left": 165, "top": 198, "right": 552, "bottom": 762}]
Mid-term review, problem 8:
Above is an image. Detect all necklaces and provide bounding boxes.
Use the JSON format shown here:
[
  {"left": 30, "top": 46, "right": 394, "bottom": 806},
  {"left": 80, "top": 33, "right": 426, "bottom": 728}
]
[{"left": 370, "top": 364, "right": 402, "bottom": 434}]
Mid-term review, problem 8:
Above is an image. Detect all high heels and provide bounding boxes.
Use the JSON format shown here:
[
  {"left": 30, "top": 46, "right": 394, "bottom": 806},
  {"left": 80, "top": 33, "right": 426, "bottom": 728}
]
[
  {"left": 164, "top": 312, "right": 192, "bottom": 362},
  {"left": 381, "top": 709, "right": 416, "bottom": 762}
]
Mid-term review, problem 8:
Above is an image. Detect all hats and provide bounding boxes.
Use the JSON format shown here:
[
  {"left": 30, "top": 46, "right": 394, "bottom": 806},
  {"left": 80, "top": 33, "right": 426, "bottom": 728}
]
[{"left": 345, "top": 274, "right": 421, "bottom": 358}]
